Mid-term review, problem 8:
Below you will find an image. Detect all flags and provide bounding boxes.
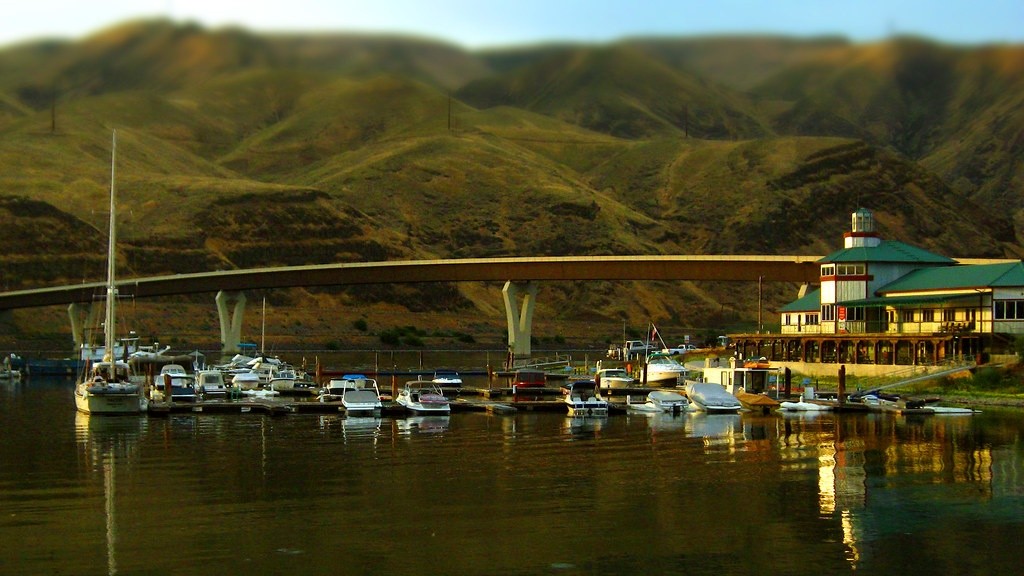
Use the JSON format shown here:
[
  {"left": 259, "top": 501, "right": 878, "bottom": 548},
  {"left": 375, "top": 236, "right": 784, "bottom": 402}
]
[{"left": 652, "top": 329, "right": 657, "bottom": 340}]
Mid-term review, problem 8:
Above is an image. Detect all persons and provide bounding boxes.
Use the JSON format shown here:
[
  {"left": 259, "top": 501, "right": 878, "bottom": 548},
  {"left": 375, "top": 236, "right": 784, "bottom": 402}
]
[{"left": 580, "top": 393, "right": 588, "bottom": 402}]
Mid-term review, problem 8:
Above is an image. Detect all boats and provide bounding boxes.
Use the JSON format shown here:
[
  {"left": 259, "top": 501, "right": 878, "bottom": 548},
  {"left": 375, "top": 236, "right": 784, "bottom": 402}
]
[
  {"left": 395, "top": 380, "right": 451, "bottom": 414},
  {"left": 647, "top": 390, "right": 690, "bottom": 414},
  {"left": 339, "top": 374, "right": 385, "bottom": 416},
  {"left": 593, "top": 367, "right": 636, "bottom": 388},
  {"left": 565, "top": 378, "right": 615, "bottom": 421},
  {"left": 685, "top": 383, "right": 742, "bottom": 414},
  {"left": 340, "top": 415, "right": 382, "bottom": 436},
  {"left": 635, "top": 320, "right": 688, "bottom": 384},
  {"left": 319, "top": 377, "right": 350, "bottom": 402},
  {"left": 564, "top": 417, "right": 606, "bottom": 427},
  {"left": 395, "top": 416, "right": 452, "bottom": 435},
  {"left": 0, "top": 352, "right": 23, "bottom": 380},
  {"left": 433, "top": 370, "right": 465, "bottom": 387},
  {"left": 67, "top": 128, "right": 315, "bottom": 413},
  {"left": 511, "top": 368, "right": 547, "bottom": 401},
  {"left": 736, "top": 392, "right": 781, "bottom": 414}
]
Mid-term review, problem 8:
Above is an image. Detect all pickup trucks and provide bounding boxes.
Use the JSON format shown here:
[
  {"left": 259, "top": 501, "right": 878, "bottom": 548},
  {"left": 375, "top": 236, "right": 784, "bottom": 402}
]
[
  {"left": 607, "top": 340, "right": 653, "bottom": 357},
  {"left": 661, "top": 344, "right": 698, "bottom": 355}
]
